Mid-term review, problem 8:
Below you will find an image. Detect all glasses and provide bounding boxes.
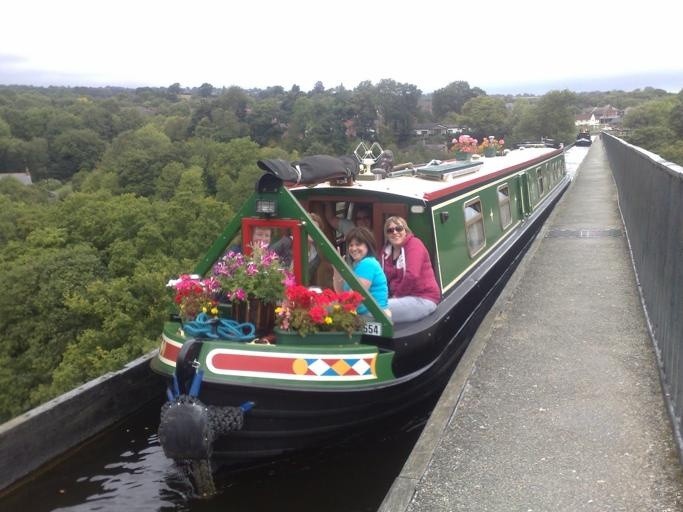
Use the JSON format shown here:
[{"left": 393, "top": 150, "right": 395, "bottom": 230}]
[{"left": 385, "top": 225, "right": 405, "bottom": 234}]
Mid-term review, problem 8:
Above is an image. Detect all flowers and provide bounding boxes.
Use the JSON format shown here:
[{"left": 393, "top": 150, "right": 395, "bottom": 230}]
[
  {"left": 286, "top": 285, "right": 366, "bottom": 341},
  {"left": 451, "top": 134, "right": 478, "bottom": 155},
  {"left": 480, "top": 134, "right": 505, "bottom": 151},
  {"left": 215, "top": 240, "right": 296, "bottom": 307}
]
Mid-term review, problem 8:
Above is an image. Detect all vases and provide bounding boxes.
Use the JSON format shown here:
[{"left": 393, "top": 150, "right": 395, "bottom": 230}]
[
  {"left": 483, "top": 148, "right": 497, "bottom": 158},
  {"left": 318, "top": 322, "right": 332, "bottom": 332},
  {"left": 455, "top": 151, "right": 473, "bottom": 161},
  {"left": 226, "top": 295, "right": 274, "bottom": 335}
]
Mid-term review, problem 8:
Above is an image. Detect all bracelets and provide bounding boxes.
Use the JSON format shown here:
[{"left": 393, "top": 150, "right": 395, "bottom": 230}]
[{"left": 331, "top": 275, "right": 346, "bottom": 283}]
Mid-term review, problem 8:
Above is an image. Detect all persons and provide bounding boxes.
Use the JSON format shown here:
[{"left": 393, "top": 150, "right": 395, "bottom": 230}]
[
  {"left": 329, "top": 225, "right": 393, "bottom": 323},
  {"left": 376, "top": 216, "right": 441, "bottom": 324},
  {"left": 212, "top": 224, "right": 273, "bottom": 306},
  {"left": 267, "top": 212, "right": 325, "bottom": 287},
  {"left": 322, "top": 200, "right": 373, "bottom": 269}
]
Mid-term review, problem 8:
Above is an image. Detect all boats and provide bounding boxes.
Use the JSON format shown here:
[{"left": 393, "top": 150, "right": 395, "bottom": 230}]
[
  {"left": 575, "top": 132, "right": 592, "bottom": 146},
  {"left": 148, "top": 137, "right": 571, "bottom": 497}
]
